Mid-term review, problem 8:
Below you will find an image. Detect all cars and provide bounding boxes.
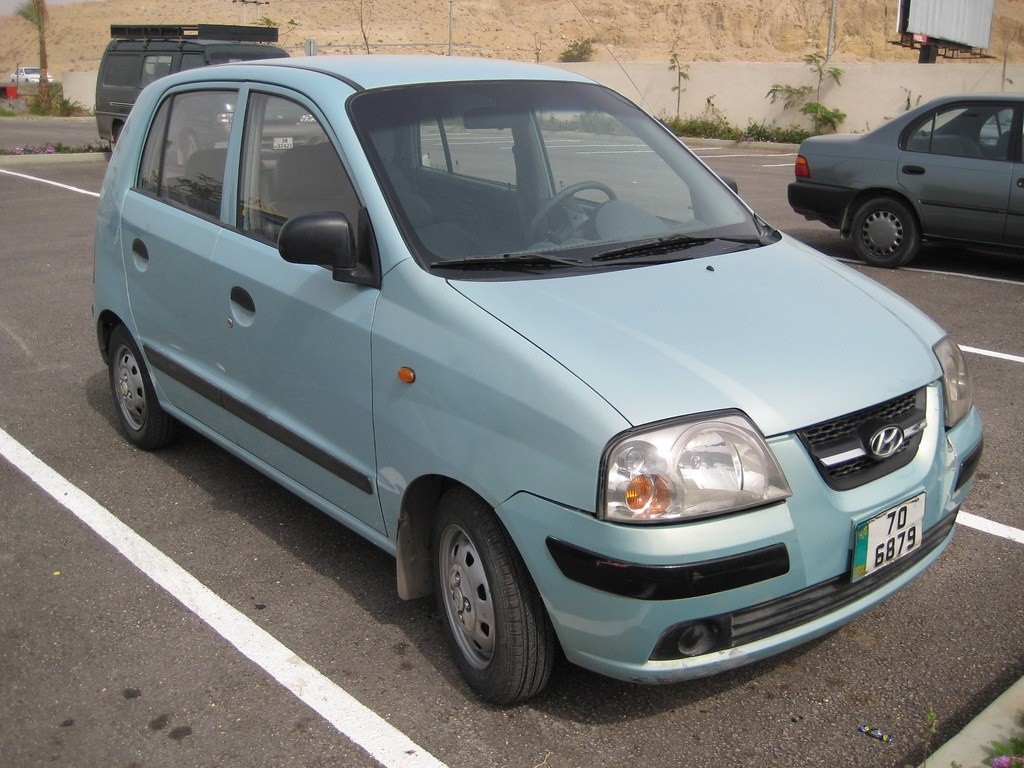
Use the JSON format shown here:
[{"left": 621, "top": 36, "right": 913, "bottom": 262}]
[
  {"left": 12, "top": 67, "right": 54, "bottom": 84},
  {"left": 92, "top": 56, "right": 983, "bottom": 705},
  {"left": 788, "top": 93, "right": 1024, "bottom": 269}
]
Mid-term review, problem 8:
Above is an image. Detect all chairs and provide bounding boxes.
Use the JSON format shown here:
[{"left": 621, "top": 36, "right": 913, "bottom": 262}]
[
  {"left": 994, "top": 130, "right": 1010, "bottom": 162},
  {"left": 269, "top": 141, "right": 361, "bottom": 239},
  {"left": 400, "top": 193, "right": 490, "bottom": 257},
  {"left": 182, "top": 147, "right": 227, "bottom": 218}
]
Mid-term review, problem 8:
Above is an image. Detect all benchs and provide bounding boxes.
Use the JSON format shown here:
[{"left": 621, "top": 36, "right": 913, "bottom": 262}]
[{"left": 910, "top": 134, "right": 986, "bottom": 158}]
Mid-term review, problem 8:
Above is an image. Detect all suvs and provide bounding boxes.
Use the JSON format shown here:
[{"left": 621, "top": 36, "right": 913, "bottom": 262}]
[{"left": 96, "top": 24, "right": 332, "bottom": 172}]
